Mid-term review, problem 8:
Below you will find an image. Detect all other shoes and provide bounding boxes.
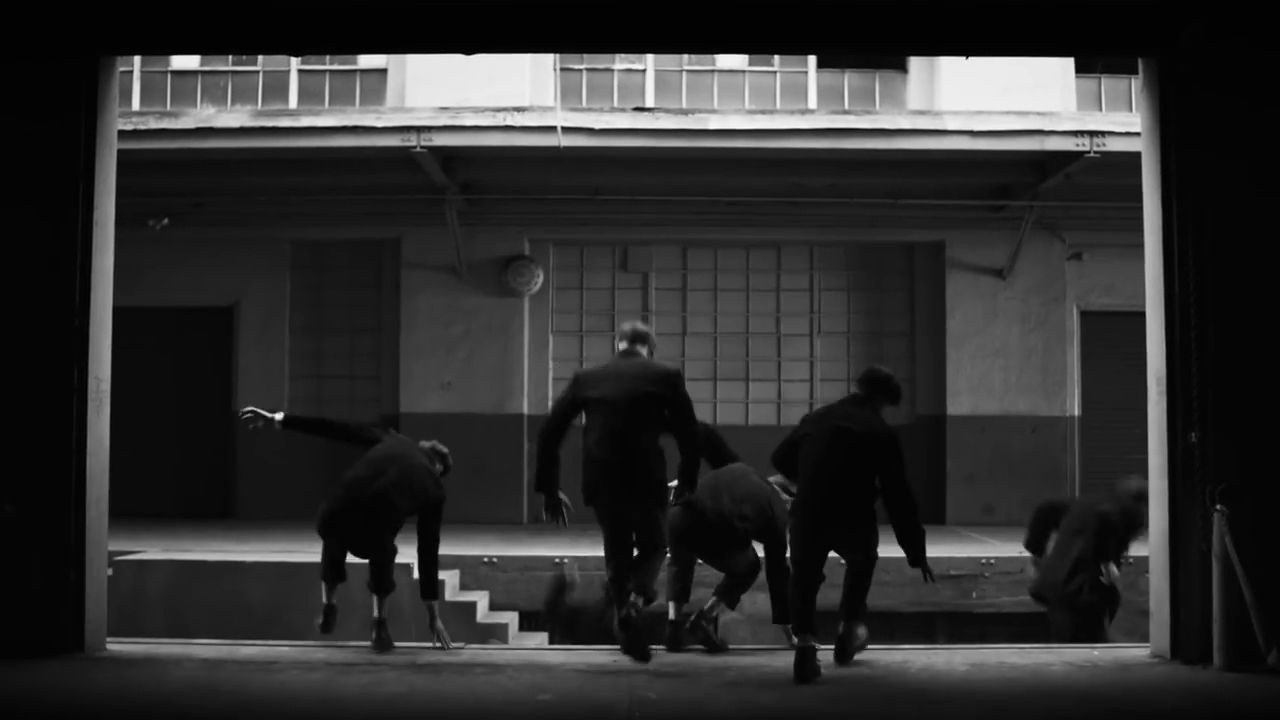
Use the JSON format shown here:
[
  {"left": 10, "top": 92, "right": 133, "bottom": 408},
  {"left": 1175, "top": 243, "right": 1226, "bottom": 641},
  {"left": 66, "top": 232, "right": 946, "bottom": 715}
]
[
  {"left": 663, "top": 619, "right": 688, "bottom": 652},
  {"left": 681, "top": 607, "right": 729, "bottom": 652},
  {"left": 614, "top": 612, "right": 654, "bottom": 663},
  {"left": 368, "top": 631, "right": 395, "bottom": 655},
  {"left": 793, "top": 643, "right": 826, "bottom": 682},
  {"left": 314, "top": 603, "right": 341, "bottom": 634},
  {"left": 833, "top": 622, "right": 869, "bottom": 664}
]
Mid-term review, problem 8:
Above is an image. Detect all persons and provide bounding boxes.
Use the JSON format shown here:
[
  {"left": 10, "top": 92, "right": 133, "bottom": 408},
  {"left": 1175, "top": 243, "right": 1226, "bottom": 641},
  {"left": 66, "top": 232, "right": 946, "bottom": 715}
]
[
  {"left": 769, "top": 367, "right": 936, "bottom": 683},
  {"left": 533, "top": 320, "right": 701, "bottom": 641},
  {"left": 665, "top": 422, "right": 797, "bottom": 651},
  {"left": 1023, "top": 474, "right": 1147, "bottom": 645},
  {"left": 239, "top": 406, "right": 455, "bottom": 651}
]
